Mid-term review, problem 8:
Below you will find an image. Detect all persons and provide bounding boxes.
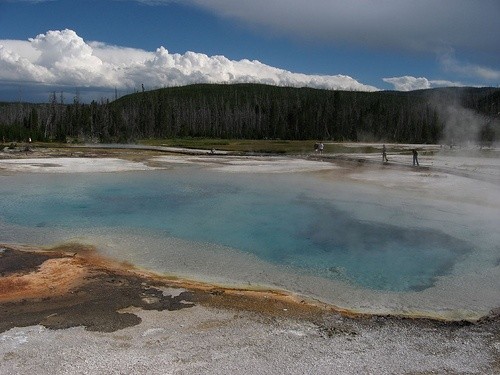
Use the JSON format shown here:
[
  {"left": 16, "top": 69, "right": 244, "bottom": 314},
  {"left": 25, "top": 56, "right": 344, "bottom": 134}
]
[
  {"left": 314, "top": 141, "right": 324, "bottom": 155},
  {"left": 408, "top": 149, "right": 421, "bottom": 166},
  {"left": 382, "top": 145, "right": 388, "bottom": 162}
]
